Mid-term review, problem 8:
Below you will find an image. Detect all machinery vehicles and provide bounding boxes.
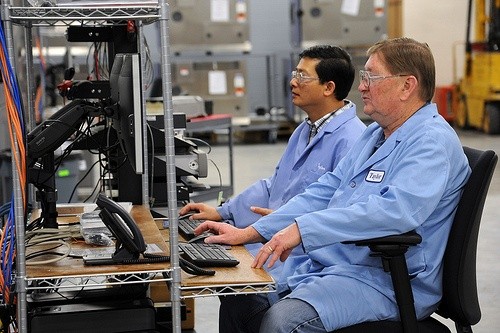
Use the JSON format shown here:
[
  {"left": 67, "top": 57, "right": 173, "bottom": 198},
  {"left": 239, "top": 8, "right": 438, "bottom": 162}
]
[{"left": 452, "top": 0, "right": 500, "bottom": 136}]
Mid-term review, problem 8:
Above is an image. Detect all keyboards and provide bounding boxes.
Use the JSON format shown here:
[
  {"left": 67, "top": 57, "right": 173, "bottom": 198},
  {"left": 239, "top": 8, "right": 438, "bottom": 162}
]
[
  {"left": 178, "top": 219, "right": 209, "bottom": 238},
  {"left": 178, "top": 243, "right": 240, "bottom": 267}
]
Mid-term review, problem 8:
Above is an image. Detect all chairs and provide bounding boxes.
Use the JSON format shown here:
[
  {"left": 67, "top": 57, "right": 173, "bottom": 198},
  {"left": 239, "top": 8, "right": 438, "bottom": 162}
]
[{"left": 329, "top": 146, "right": 498, "bottom": 333}]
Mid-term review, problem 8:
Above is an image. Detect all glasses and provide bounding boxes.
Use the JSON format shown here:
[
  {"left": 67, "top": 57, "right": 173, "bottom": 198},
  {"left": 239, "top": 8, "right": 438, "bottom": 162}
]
[
  {"left": 292, "top": 70, "right": 326, "bottom": 84},
  {"left": 358, "top": 69, "right": 418, "bottom": 87}
]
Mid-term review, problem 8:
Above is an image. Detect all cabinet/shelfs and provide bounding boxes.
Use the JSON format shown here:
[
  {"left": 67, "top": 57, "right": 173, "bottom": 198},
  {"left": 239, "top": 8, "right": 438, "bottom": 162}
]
[{"left": 6, "top": 0, "right": 276, "bottom": 333}]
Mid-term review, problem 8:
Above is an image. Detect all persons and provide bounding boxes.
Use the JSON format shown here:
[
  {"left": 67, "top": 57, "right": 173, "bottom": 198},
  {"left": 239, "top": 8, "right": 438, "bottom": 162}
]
[
  {"left": 179, "top": 44, "right": 367, "bottom": 270},
  {"left": 194, "top": 37, "right": 471, "bottom": 333}
]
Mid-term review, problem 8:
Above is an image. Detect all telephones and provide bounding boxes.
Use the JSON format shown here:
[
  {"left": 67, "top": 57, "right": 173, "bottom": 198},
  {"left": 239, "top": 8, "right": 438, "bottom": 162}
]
[{"left": 96, "top": 193, "right": 147, "bottom": 266}]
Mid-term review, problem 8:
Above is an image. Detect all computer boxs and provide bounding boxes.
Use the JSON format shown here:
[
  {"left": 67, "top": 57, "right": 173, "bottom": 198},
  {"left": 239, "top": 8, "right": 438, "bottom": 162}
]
[{"left": 26, "top": 287, "right": 156, "bottom": 333}]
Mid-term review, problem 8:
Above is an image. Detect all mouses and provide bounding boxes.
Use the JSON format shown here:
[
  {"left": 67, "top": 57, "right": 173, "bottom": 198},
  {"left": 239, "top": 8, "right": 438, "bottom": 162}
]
[
  {"left": 178, "top": 213, "right": 193, "bottom": 220},
  {"left": 187, "top": 232, "right": 215, "bottom": 244}
]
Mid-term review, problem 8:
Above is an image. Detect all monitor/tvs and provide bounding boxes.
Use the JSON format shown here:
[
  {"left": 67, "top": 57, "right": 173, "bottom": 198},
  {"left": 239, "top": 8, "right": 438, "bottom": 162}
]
[{"left": 109, "top": 53, "right": 145, "bottom": 175}]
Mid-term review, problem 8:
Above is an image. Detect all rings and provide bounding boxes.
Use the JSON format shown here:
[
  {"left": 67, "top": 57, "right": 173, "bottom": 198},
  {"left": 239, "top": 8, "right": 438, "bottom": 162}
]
[{"left": 269, "top": 246, "right": 273, "bottom": 251}]
